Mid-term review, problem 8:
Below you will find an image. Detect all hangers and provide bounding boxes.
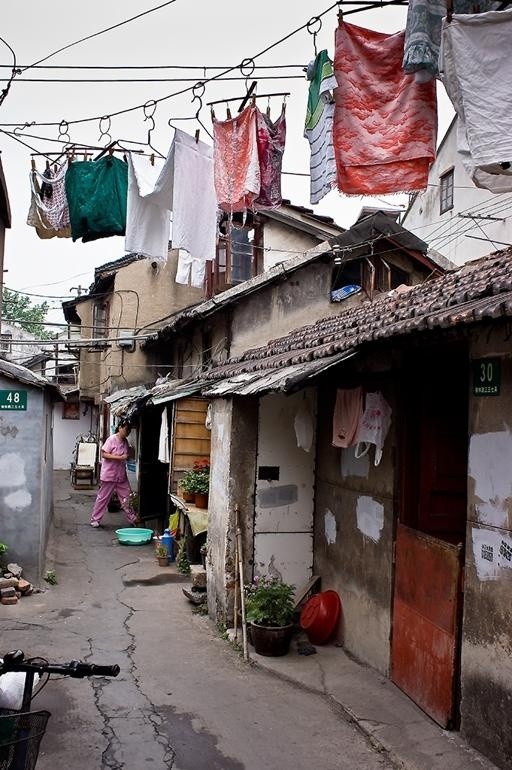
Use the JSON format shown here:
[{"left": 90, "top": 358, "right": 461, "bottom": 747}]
[
  {"left": 168, "top": 97, "right": 215, "bottom": 143},
  {"left": 118, "top": 116, "right": 165, "bottom": 160},
  {"left": 313, "top": 31, "right": 319, "bottom": 57}
]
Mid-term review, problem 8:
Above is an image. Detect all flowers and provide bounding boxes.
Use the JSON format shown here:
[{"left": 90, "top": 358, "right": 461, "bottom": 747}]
[
  {"left": 244, "top": 577, "right": 275, "bottom": 595},
  {"left": 178, "top": 459, "right": 210, "bottom": 495}
]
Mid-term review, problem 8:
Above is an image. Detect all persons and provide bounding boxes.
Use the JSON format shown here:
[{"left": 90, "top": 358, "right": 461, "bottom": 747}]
[{"left": 90, "top": 420, "right": 140, "bottom": 529}]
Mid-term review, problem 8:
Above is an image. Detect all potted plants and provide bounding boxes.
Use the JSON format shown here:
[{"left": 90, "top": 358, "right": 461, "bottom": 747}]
[
  {"left": 157, "top": 547, "right": 170, "bottom": 566},
  {"left": 247, "top": 575, "right": 294, "bottom": 657},
  {"left": 108, "top": 493, "right": 121, "bottom": 513}
]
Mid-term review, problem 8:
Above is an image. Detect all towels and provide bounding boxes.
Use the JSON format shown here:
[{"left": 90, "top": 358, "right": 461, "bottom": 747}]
[{"left": 331, "top": 386, "right": 363, "bottom": 448}]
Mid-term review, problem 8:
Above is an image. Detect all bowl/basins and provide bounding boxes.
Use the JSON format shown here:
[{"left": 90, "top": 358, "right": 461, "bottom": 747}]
[
  {"left": 299, "top": 589, "right": 341, "bottom": 646},
  {"left": 115, "top": 527, "right": 155, "bottom": 546}
]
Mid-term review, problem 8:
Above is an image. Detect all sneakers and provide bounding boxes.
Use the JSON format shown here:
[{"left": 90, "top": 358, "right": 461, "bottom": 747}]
[{"left": 90, "top": 521, "right": 100, "bottom": 528}]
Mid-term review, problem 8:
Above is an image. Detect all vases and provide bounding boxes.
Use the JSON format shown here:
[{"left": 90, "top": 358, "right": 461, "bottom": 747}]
[
  {"left": 183, "top": 491, "right": 194, "bottom": 503},
  {"left": 238, "top": 608, "right": 254, "bottom": 642},
  {"left": 195, "top": 492, "right": 208, "bottom": 508}
]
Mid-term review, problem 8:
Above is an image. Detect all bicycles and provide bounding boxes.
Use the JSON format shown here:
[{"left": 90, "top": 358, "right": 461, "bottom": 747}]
[{"left": 0, "top": 649, "right": 120, "bottom": 770}]
